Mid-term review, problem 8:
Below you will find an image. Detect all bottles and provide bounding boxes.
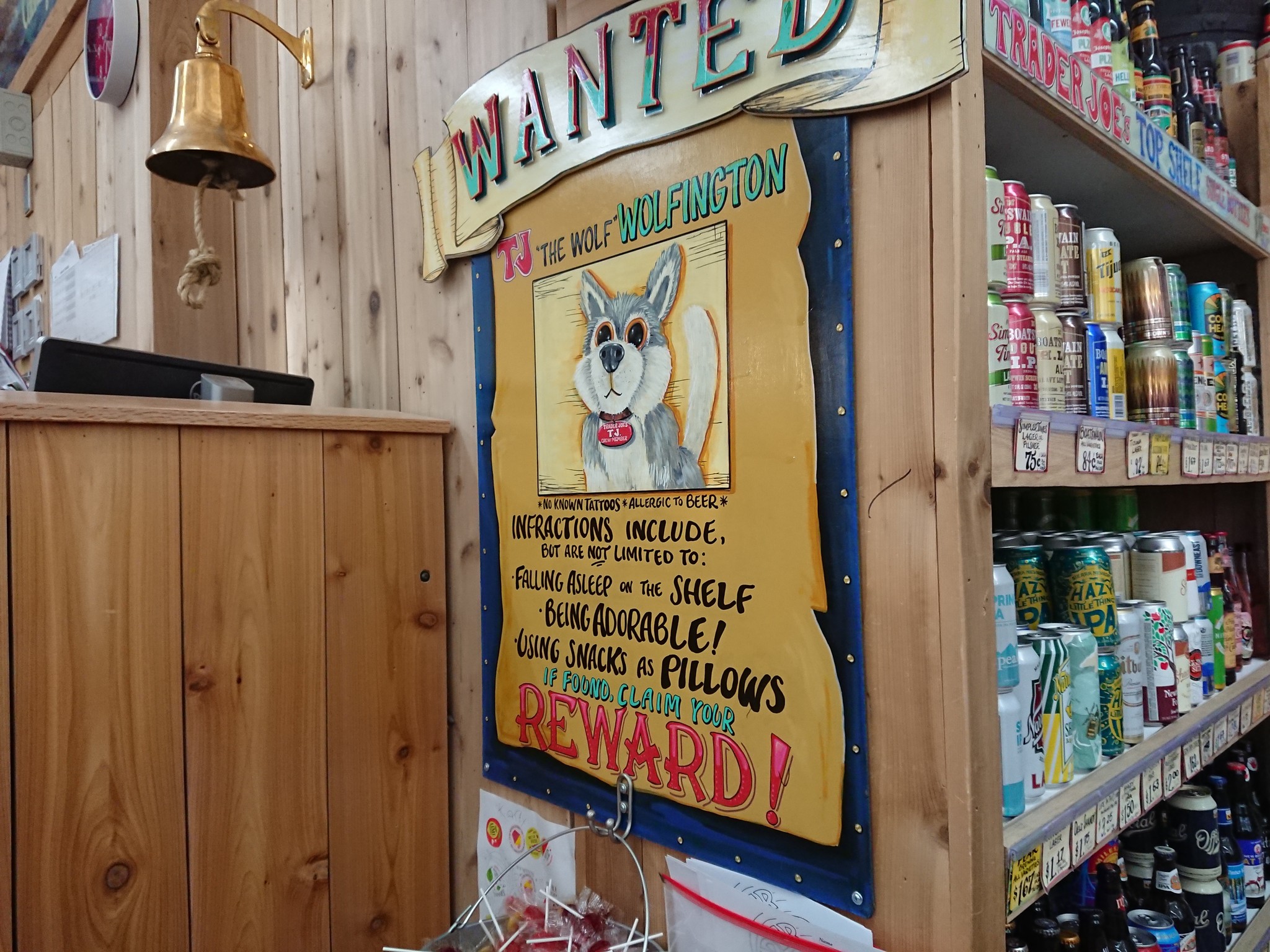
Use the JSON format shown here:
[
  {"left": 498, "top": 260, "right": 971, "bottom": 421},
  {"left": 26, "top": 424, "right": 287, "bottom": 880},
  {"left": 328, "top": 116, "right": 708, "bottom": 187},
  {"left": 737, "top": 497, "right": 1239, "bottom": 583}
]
[
  {"left": 1095, "top": 863, "right": 1139, "bottom": 952},
  {"left": 1237, "top": 740, "right": 1258, "bottom": 790},
  {"left": 1229, "top": 749, "right": 1270, "bottom": 880},
  {"left": 1227, "top": 539, "right": 1253, "bottom": 665},
  {"left": 1006, "top": 936, "right": 1030, "bottom": 952},
  {"left": 1209, "top": 775, "right": 1247, "bottom": 933},
  {"left": 1167, "top": 43, "right": 1205, "bottom": 164},
  {"left": 1103, "top": 0, "right": 1136, "bottom": 107},
  {"left": 1203, "top": 531, "right": 1243, "bottom": 673},
  {"left": 1228, "top": 138, "right": 1239, "bottom": 192},
  {"left": 1214, "top": 84, "right": 1227, "bottom": 128},
  {"left": 1129, "top": 0, "right": 1172, "bottom": 137},
  {"left": 1134, "top": 51, "right": 1144, "bottom": 112},
  {"left": 1225, "top": 761, "right": 1265, "bottom": 908},
  {"left": 1199, "top": 67, "right": 1228, "bottom": 184},
  {"left": 1088, "top": 0, "right": 1113, "bottom": 87},
  {"left": 1050, "top": 0, "right": 1072, "bottom": 51},
  {"left": 1071, "top": 0, "right": 1091, "bottom": 69},
  {"left": 1189, "top": 56, "right": 1215, "bottom": 174},
  {"left": 1057, "top": 931, "right": 1081, "bottom": 952},
  {"left": 1031, "top": 919, "right": 1059, "bottom": 952},
  {"left": 1005, "top": 920, "right": 1019, "bottom": 936},
  {"left": 1149, "top": 846, "right": 1198, "bottom": 952},
  {"left": 1078, "top": 906, "right": 1110, "bottom": 952}
]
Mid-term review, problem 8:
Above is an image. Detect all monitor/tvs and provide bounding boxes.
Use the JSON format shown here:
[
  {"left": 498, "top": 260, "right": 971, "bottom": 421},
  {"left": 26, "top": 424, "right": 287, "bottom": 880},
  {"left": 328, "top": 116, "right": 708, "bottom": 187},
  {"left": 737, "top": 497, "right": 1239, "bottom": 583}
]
[{"left": 26, "top": 336, "right": 315, "bottom": 406}]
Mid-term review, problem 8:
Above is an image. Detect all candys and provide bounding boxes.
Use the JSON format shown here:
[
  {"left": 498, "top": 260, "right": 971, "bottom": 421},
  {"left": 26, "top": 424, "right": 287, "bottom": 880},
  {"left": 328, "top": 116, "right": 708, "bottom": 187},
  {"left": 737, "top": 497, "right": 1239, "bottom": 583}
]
[{"left": 382, "top": 879, "right": 663, "bottom": 952}]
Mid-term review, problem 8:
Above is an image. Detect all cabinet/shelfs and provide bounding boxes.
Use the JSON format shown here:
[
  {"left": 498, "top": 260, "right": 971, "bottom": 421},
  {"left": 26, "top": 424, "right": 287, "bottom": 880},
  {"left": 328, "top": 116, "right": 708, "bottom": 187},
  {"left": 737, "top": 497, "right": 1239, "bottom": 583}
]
[{"left": 471, "top": 0, "right": 1270, "bottom": 952}]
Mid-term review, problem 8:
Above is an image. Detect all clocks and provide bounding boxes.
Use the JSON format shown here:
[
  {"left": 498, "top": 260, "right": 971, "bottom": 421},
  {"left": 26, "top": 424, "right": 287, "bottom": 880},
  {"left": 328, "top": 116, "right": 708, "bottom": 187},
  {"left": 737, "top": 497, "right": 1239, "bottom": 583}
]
[{"left": 83, "top": 0, "right": 139, "bottom": 107}]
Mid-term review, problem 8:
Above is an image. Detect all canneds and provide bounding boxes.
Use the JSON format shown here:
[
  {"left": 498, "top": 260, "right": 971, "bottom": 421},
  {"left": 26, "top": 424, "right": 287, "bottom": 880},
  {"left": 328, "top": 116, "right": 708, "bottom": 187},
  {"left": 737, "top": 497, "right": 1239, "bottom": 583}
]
[
  {"left": 984, "top": 165, "right": 1262, "bottom": 439},
  {"left": 1034, "top": 784, "right": 1228, "bottom": 952},
  {"left": 991, "top": 525, "right": 1227, "bottom": 818},
  {"left": 1216, "top": 34, "right": 1270, "bottom": 88}
]
